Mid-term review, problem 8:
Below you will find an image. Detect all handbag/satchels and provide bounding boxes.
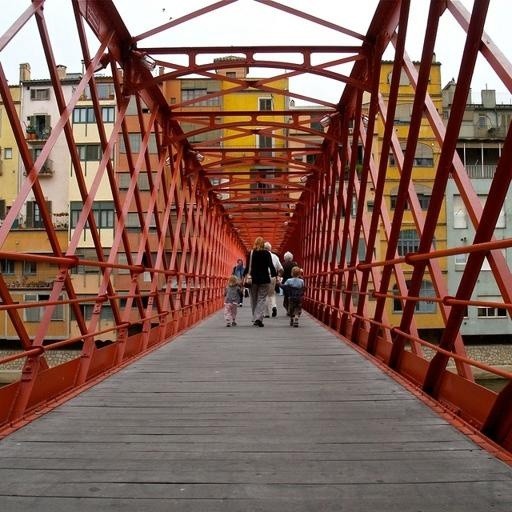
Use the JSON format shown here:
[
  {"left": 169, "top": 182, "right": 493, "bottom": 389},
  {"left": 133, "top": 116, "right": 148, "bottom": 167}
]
[{"left": 243, "top": 272, "right": 253, "bottom": 288}]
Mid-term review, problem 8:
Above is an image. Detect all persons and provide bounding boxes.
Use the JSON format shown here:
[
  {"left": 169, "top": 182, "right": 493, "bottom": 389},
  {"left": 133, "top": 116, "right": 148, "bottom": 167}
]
[
  {"left": 279, "top": 266, "right": 305, "bottom": 327},
  {"left": 231, "top": 259, "right": 245, "bottom": 307},
  {"left": 263, "top": 241, "right": 285, "bottom": 317},
  {"left": 222, "top": 274, "right": 244, "bottom": 326},
  {"left": 242, "top": 236, "right": 279, "bottom": 327},
  {"left": 279, "top": 250, "right": 298, "bottom": 316}
]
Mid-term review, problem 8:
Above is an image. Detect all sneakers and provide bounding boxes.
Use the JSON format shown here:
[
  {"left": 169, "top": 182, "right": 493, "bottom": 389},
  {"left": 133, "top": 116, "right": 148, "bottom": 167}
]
[
  {"left": 290, "top": 316, "right": 299, "bottom": 328},
  {"left": 237, "top": 303, "right": 243, "bottom": 308},
  {"left": 226, "top": 321, "right": 238, "bottom": 327},
  {"left": 251, "top": 319, "right": 264, "bottom": 328},
  {"left": 263, "top": 315, "right": 270, "bottom": 318},
  {"left": 272, "top": 306, "right": 277, "bottom": 317}
]
